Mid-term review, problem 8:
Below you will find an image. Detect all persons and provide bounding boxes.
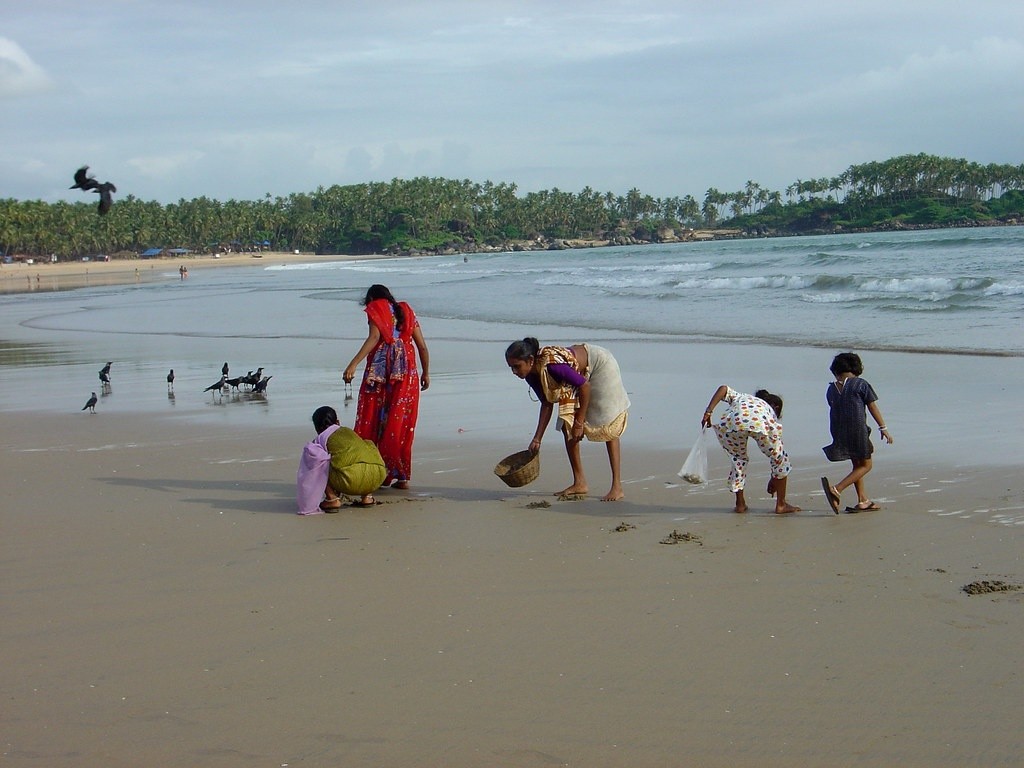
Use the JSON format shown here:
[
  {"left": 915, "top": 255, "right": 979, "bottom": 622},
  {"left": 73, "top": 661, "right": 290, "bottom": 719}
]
[
  {"left": 296, "top": 406, "right": 387, "bottom": 516},
  {"left": 135, "top": 268, "right": 139, "bottom": 279},
  {"left": 701, "top": 385, "right": 801, "bottom": 514},
  {"left": 179, "top": 266, "right": 187, "bottom": 281},
  {"left": 37, "top": 274, "right": 39, "bottom": 282},
  {"left": 821, "top": 353, "right": 893, "bottom": 515},
  {"left": 505, "top": 337, "right": 631, "bottom": 502},
  {"left": 342, "top": 284, "right": 430, "bottom": 489}
]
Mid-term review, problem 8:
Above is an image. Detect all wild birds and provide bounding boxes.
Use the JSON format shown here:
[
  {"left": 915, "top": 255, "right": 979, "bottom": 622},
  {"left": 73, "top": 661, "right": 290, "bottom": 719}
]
[
  {"left": 167, "top": 368, "right": 174, "bottom": 388},
  {"left": 81, "top": 391, "right": 97, "bottom": 413},
  {"left": 203, "top": 362, "right": 273, "bottom": 395},
  {"left": 68, "top": 165, "right": 116, "bottom": 217},
  {"left": 98, "top": 361, "right": 113, "bottom": 386}
]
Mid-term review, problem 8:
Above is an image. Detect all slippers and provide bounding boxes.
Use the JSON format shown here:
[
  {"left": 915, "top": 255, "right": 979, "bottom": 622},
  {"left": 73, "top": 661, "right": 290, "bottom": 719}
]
[
  {"left": 319, "top": 501, "right": 339, "bottom": 513},
  {"left": 362, "top": 498, "right": 376, "bottom": 508},
  {"left": 845, "top": 502, "right": 880, "bottom": 512},
  {"left": 820, "top": 476, "right": 841, "bottom": 515}
]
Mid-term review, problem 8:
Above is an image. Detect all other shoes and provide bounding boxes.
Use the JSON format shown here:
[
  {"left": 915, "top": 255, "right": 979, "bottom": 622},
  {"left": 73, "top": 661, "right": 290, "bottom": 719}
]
[{"left": 391, "top": 481, "right": 409, "bottom": 489}]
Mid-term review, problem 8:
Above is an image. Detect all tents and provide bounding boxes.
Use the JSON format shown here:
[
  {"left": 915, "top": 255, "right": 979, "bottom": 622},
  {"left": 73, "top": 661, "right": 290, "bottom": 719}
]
[{"left": 139, "top": 248, "right": 193, "bottom": 260}]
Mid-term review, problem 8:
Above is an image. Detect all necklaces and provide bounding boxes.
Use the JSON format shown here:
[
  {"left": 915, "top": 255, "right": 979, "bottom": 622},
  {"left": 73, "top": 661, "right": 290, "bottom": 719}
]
[{"left": 528, "top": 382, "right": 543, "bottom": 402}]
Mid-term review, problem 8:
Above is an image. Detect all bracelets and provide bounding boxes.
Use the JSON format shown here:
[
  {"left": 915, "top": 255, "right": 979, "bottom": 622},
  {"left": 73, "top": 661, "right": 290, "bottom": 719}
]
[
  {"left": 532, "top": 440, "right": 541, "bottom": 444},
  {"left": 575, "top": 418, "right": 586, "bottom": 428},
  {"left": 704, "top": 408, "right": 713, "bottom": 415},
  {"left": 878, "top": 425, "right": 887, "bottom": 431}
]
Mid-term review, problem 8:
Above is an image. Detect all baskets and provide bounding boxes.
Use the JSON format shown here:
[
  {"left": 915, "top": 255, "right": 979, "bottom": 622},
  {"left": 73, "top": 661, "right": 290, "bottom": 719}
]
[{"left": 493, "top": 449, "right": 540, "bottom": 488}]
